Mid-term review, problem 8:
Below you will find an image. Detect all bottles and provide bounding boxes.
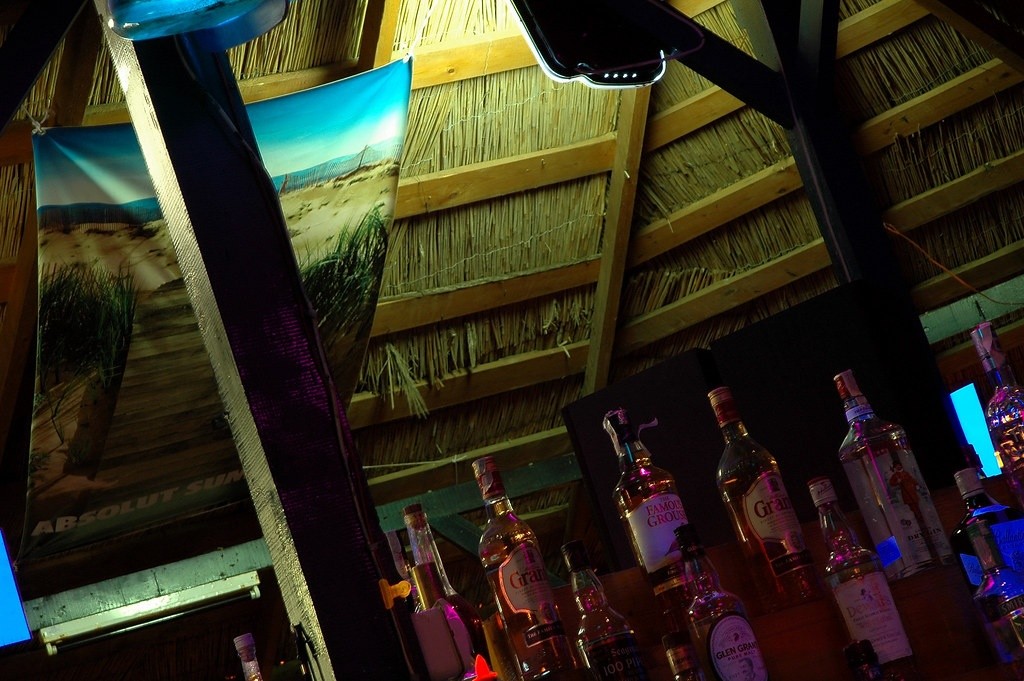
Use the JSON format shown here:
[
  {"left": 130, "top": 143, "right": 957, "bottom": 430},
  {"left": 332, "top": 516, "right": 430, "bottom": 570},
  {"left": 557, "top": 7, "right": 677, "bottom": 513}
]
[
  {"left": 971, "top": 322, "right": 1023, "bottom": 493},
  {"left": 400, "top": 501, "right": 490, "bottom": 678},
  {"left": 844, "top": 638, "right": 886, "bottom": 681},
  {"left": 234, "top": 632, "right": 265, "bottom": 681},
  {"left": 660, "top": 630, "right": 706, "bottom": 681},
  {"left": 806, "top": 475, "right": 921, "bottom": 681},
  {"left": 603, "top": 407, "right": 717, "bottom": 627},
  {"left": 962, "top": 520, "right": 1024, "bottom": 670},
  {"left": 830, "top": 369, "right": 954, "bottom": 579},
  {"left": 560, "top": 540, "right": 653, "bottom": 681},
  {"left": 704, "top": 387, "right": 822, "bottom": 612},
  {"left": 471, "top": 455, "right": 575, "bottom": 680},
  {"left": 672, "top": 521, "right": 769, "bottom": 681}
]
